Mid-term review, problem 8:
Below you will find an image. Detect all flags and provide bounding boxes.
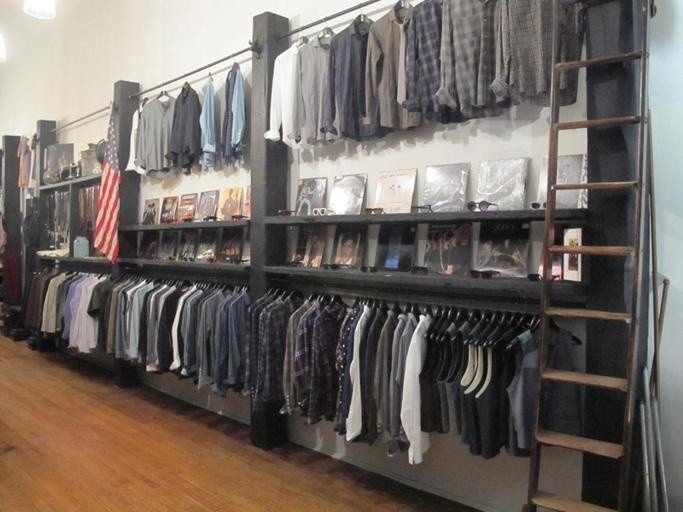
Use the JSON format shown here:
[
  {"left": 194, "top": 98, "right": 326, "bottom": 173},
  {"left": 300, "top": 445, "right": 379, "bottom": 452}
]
[{"left": 91, "top": 99, "right": 122, "bottom": 267}]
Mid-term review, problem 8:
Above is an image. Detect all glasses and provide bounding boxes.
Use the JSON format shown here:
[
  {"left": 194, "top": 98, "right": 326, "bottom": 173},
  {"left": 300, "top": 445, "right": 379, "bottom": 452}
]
[
  {"left": 163, "top": 219, "right": 177, "bottom": 225},
  {"left": 359, "top": 264, "right": 379, "bottom": 276},
  {"left": 363, "top": 207, "right": 386, "bottom": 216},
  {"left": 409, "top": 204, "right": 432, "bottom": 213},
  {"left": 182, "top": 217, "right": 198, "bottom": 224},
  {"left": 230, "top": 214, "right": 250, "bottom": 222},
  {"left": 321, "top": 262, "right": 343, "bottom": 272},
  {"left": 525, "top": 272, "right": 561, "bottom": 281},
  {"left": 312, "top": 207, "right": 332, "bottom": 214},
  {"left": 469, "top": 269, "right": 501, "bottom": 278},
  {"left": 528, "top": 198, "right": 548, "bottom": 211},
  {"left": 466, "top": 200, "right": 501, "bottom": 211},
  {"left": 135, "top": 222, "right": 145, "bottom": 226},
  {"left": 273, "top": 209, "right": 299, "bottom": 215},
  {"left": 206, "top": 257, "right": 218, "bottom": 264},
  {"left": 168, "top": 256, "right": 177, "bottom": 262},
  {"left": 410, "top": 266, "right": 430, "bottom": 274},
  {"left": 284, "top": 259, "right": 306, "bottom": 268},
  {"left": 203, "top": 216, "right": 218, "bottom": 222},
  {"left": 183, "top": 257, "right": 195, "bottom": 262}
]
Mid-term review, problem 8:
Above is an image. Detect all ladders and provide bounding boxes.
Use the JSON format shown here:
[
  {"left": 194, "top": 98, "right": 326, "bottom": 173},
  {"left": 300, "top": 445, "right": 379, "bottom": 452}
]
[{"left": 525, "top": 0, "right": 648, "bottom": 512}]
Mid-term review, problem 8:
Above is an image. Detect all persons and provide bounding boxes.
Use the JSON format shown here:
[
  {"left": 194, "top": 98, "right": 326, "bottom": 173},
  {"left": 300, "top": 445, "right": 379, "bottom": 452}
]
[
  {"left": 296, "top": 181, "right": 315, "bottom": 216},
  {"left": 338, "top": 232, "right": 356, "bottom": 265}
]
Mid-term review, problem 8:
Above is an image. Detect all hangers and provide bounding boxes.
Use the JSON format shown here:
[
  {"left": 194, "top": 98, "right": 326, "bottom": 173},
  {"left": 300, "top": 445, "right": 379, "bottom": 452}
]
[
  {"left": 355, "top": 3, "right": 368, "bottom": 24},
  {"left": 40, "top": 261, "right": 111, "bottom": 281},
  {"left": 395, "top": 0, "right": 412, "bottom": 9},
  {"left": 207, "top": 64, "right": 214, "bottom": 82},
  {"left": 141, "top": 89, "right": 149, "bottom": 107},
  {"left": 157, "top": 83, "right": 170, "bottom": 100},
  {"left": 230, "top": 53, "right": 238, "bottom": 71},
  {"left": 319, "top": 17, "right": 332, "bottom": 37},
  {"left": 121, "top": 272, "right": 249, "bottom": 296},
  {"left": 263, "top": 286, "right": 557, "bottom": 334},
  {"left": 182, "top": 74, "right": 189, "bottom": 93},
  {"left": 296, "top": 25, "right": 309, "bottom": 47}
]
